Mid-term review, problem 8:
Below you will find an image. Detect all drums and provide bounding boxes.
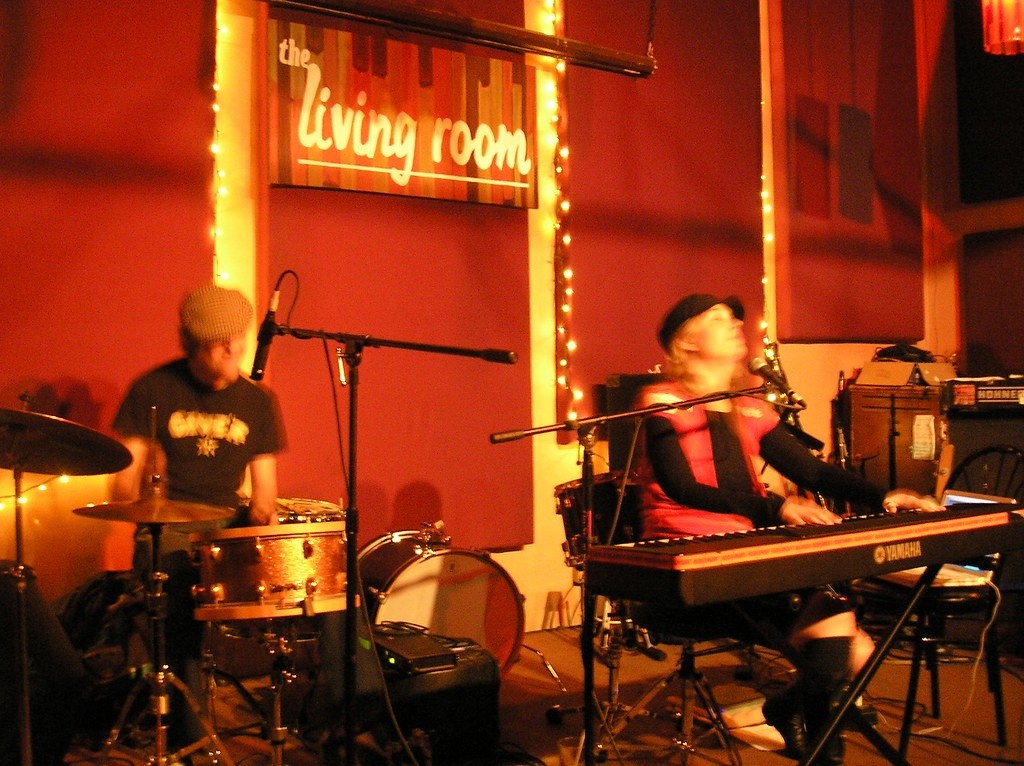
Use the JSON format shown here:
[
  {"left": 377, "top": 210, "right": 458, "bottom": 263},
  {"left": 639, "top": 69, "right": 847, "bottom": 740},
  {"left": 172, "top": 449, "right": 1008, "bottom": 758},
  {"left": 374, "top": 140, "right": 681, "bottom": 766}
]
[
  {"left": 552, "top": 470, "right": 643, "bottom": 574},
  {"left": 219, "top": 494, "right": 348, "bottom": 644},
  {"left": 356, "top": 527, "right": 527, "bottom": 684},
  {"left": 185, "top": 519, "right": 362, "bottom": 624}
]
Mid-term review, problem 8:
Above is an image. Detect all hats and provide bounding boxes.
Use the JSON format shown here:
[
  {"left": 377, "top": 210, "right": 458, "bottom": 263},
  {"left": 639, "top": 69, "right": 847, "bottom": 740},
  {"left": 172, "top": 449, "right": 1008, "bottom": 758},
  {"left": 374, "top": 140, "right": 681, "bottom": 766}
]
[
  {"left": 658, "top": 293, "right": 745, "bottom": 356},
  {"left": 178, "top": 284, "right": 252, "bottom": 346}
]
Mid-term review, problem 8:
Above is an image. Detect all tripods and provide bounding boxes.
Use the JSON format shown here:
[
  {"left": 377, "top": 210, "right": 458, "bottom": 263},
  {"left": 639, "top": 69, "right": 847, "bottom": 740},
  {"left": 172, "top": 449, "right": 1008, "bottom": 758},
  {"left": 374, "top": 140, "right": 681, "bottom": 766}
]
[{"left": 94, "top": 524, "right": 235, "bottom": 766}]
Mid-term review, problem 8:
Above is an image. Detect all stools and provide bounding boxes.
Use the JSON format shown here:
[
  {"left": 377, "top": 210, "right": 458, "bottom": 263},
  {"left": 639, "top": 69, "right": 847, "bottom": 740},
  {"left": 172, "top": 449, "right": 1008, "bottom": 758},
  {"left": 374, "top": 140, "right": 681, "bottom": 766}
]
[{"left": 615, "top": 599, "right": 757, "bottom": 766}]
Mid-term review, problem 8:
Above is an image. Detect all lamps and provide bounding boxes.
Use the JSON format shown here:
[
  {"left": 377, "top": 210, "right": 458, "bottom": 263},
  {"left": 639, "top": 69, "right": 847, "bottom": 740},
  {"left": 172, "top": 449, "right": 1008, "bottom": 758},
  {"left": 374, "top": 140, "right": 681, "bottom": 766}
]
[{"left": 268, "top": 0, "right": 661, "bottom": 79}]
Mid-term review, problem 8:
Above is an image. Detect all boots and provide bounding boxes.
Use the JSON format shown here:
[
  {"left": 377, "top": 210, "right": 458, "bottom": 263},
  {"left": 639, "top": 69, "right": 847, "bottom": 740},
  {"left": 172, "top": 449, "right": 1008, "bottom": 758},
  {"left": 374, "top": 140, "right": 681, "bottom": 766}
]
[
  {"left": 799, "top": 637, "right": 845, "bottom": 766},
  {"left": 762, "top": 675, "right": 854, "bottom": 760}
]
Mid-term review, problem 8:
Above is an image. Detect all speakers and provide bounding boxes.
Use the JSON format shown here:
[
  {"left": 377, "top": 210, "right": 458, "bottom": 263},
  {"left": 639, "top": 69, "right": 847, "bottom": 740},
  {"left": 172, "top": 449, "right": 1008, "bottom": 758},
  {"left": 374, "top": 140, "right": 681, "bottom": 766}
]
[{"left": 366, "top": 631, "right": 502, "bottom": 766}]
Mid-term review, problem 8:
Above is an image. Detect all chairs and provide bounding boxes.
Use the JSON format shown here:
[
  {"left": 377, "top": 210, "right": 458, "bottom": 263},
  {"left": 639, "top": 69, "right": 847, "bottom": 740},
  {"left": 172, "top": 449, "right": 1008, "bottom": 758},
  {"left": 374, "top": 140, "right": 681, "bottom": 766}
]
[{"left": 848, "top": 441, "right": 1023, "bottom": 765}]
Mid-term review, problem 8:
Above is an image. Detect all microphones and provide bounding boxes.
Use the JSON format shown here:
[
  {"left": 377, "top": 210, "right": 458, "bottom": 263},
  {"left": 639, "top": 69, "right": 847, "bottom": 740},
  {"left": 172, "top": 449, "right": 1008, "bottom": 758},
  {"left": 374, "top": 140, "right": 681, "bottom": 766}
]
[
  {"left": 249, "top": 311, "right": 275, "bottom": 382},
  {"left": 747, "top": 356, "right": 802, "bottom": 405}
]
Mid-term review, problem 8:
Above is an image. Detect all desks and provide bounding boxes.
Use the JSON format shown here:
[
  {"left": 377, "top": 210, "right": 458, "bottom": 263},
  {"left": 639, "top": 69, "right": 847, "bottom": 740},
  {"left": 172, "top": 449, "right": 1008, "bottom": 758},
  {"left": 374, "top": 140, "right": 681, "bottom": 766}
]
[{"left": 846, "top": 383, "right": 1024, "bottom": 502}]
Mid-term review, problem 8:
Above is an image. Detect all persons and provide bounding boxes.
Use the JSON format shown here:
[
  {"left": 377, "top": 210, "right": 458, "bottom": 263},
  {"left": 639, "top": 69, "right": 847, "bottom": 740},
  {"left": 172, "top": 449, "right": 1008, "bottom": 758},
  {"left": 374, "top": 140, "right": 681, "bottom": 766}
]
[
  {"left": 107, "top": 282, "right": 290, "bottom": 754},
  {"left": 629, "top": 291, "right": 949, "bottom": 765}
]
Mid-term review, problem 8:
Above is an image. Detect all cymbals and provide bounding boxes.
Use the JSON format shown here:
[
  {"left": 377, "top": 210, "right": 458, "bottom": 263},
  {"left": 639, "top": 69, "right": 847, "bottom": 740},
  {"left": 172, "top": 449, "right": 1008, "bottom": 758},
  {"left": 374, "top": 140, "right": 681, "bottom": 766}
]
[
  {"left": 0, "top": 388, "right": 135, "bottom": 479},
  {"left": 72, "top": 482, "right": 238, "bottom": 525}
]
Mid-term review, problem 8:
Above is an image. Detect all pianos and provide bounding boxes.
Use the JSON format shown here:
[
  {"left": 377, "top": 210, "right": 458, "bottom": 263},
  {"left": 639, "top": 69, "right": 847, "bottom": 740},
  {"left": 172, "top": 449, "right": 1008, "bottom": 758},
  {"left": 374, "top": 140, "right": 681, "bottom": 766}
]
[{"left": 585, "top": 500, "right": 1024, "bottom": 611}]
